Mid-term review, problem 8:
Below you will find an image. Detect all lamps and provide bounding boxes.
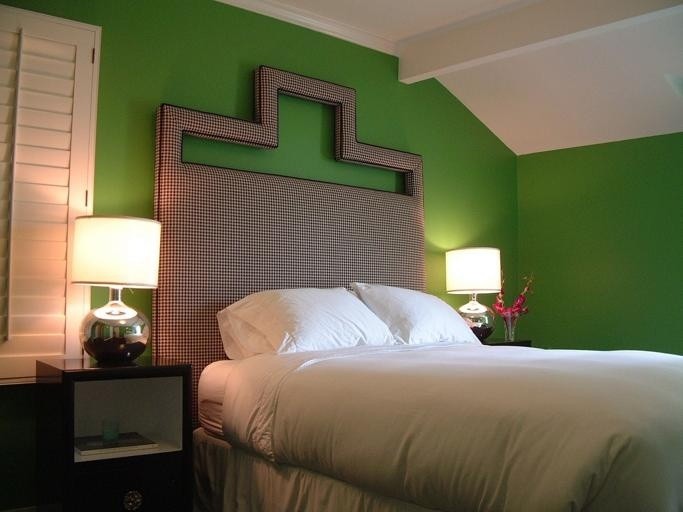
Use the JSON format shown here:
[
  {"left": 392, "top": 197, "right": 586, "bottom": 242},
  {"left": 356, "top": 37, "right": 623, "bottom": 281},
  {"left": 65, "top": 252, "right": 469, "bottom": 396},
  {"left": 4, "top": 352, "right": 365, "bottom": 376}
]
[
  {"left": 444, "top": 247, "right": 506, "bottom": 342},
  {"left": 63, "top": 212, "right": 166, "bottom": 368}
]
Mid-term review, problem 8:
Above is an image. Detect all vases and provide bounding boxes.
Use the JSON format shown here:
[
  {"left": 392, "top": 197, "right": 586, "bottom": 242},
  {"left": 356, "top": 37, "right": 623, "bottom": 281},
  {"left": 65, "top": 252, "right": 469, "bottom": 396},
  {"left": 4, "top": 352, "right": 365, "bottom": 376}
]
[{"left": 499, "top": 311, "right": 519, "bottom": 341}]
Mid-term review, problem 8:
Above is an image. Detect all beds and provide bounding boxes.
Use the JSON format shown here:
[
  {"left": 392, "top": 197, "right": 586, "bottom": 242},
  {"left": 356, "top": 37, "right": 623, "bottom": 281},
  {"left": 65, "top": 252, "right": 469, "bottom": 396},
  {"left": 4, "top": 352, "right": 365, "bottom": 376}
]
[{"left": 145, "top": 64, "right": 681, "bottom": 511}]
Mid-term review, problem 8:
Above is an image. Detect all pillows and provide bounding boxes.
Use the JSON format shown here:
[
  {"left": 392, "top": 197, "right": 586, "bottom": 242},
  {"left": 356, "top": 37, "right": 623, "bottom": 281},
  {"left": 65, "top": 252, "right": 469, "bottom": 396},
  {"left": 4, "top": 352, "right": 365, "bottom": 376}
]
[{"left": 209, "top": 283, "right": 483, "bottom": 362}]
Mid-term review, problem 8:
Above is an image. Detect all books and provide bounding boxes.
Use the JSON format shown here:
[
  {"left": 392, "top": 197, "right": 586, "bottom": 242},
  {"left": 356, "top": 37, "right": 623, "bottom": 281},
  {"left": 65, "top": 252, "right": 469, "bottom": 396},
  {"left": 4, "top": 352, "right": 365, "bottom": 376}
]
[{"left": 74, "top": 431, "right": 159, "bottom": 456}]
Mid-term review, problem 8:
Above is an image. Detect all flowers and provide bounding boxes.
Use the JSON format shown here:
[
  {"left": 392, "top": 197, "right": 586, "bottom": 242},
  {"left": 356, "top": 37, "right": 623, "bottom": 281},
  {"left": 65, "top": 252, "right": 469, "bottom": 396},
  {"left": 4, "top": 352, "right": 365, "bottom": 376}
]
[{"left": 490, "top": 269, "right": 536, "bottom": 321}]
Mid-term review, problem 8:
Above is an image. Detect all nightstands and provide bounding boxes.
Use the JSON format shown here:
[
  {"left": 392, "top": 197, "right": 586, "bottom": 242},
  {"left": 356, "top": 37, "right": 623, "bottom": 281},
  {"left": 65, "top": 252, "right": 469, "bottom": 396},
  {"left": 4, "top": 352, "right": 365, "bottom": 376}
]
[
  {"left": 481, "top": 337, "right": 532, "bottom": 347},
  {"left": 30, "top": 355, "right": 196, "bottom": 510}
]
